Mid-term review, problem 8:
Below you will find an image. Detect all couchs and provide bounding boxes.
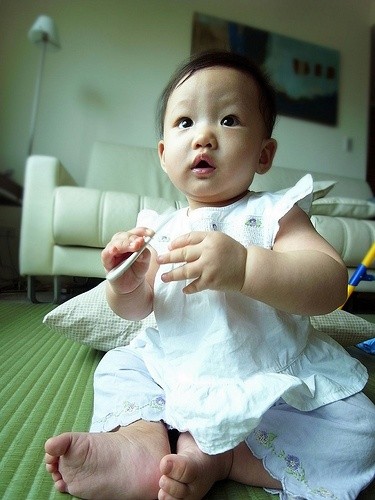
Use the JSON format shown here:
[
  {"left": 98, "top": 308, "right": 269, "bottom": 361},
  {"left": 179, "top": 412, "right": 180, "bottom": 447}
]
[{"left": 18, "top": 142, "right": 375, "bottom": 305}]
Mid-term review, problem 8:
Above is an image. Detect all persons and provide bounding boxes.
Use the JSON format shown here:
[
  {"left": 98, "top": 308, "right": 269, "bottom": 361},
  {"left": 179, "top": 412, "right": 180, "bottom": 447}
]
[{"left": 41, "top": 48, "right": 375, "bottom": 500}]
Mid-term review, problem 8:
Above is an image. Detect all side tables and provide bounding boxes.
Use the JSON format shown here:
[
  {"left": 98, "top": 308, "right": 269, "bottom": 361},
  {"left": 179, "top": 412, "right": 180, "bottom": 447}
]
[{"left": 0, "top": 176, "right": 23, "bottom": 291}]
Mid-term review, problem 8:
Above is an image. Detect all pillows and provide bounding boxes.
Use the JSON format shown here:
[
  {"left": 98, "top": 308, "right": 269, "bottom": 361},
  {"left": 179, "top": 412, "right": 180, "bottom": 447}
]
[
  {"left": 274, "top": 181, "right": 337, "bottom": 201},
  {"left": 311, "top": 197, "right": 375, "bottom": 219},
  {"left": 42, "top": 279, "right": 375, "bottom": 352}
]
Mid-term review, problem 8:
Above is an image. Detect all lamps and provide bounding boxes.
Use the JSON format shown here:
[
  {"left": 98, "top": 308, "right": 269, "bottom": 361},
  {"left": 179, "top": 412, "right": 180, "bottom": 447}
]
[{"left": 25, "top": 15, "right": 62, "bottom": 158}]
